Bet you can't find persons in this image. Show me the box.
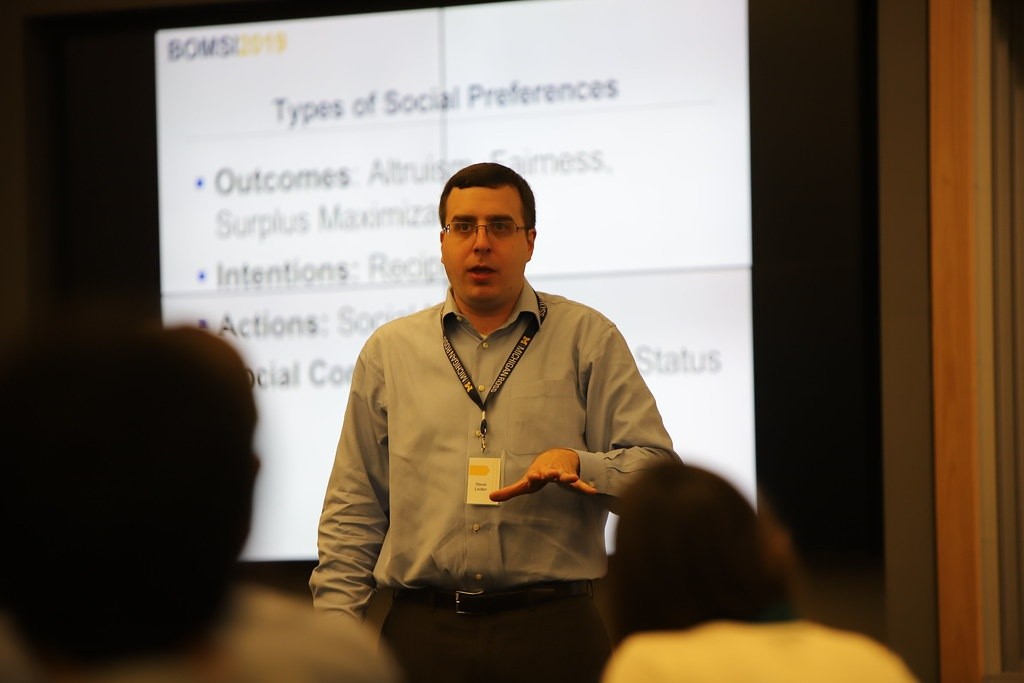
[604,463,915,683]
[309,163,681,683]
[1,299,404,683]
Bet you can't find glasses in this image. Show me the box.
[443,218,533,239]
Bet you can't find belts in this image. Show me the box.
[394,583,588,617]
